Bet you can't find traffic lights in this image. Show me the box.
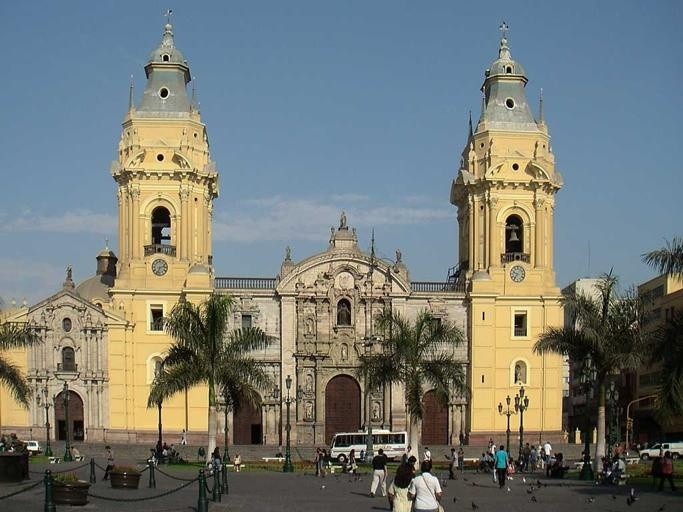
[628,418,633,428]
[654,400,659,409]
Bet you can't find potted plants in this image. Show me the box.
[47,470,92,506]
[105,462,143,490]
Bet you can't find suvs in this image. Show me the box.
[21,440,43,457]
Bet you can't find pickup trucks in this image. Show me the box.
[639,440,682,460]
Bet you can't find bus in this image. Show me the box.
[327,428,411,464]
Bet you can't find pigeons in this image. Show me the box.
[471,502,480,511]
[583,488,642,506]
[507,485,512,493]
[521,475,550,503]
[453,496,458,503]
[473,482,479,487]
[657,504,667,512]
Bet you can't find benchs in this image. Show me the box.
[261,456,285,463]
[225,463,245,472]
[574,459,593,471]
[461,456,480,465]
[330,464,358,475]
[625,457,641,465]
[595,468,629,486]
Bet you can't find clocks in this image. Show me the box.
[509,265,526,283]
[151,259,168,276]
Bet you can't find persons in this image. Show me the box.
[369,449,388,498]
[233,449,243,473]
[71,445,81,455]
[479,437,552,488]
[178,428,187,446]
[315,447,330,474]
[658,451,679,492]
[601,441,647,487]
[210,447,222,473]
[651,450,664,490]
[0,433,29,456]
[342,449,364,474]
[443,447,465,480]
[102,446,115,480]
[148,439,184,467]
[388,445,443,512]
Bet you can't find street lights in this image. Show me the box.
[153,361,167,465]
[605,378,620,459]
[214,382,239,462]
[497,393,517,458]
[34,386,58,457]
[272,372,307,473]
[515,385,531,459]
[60,381,75,461]
